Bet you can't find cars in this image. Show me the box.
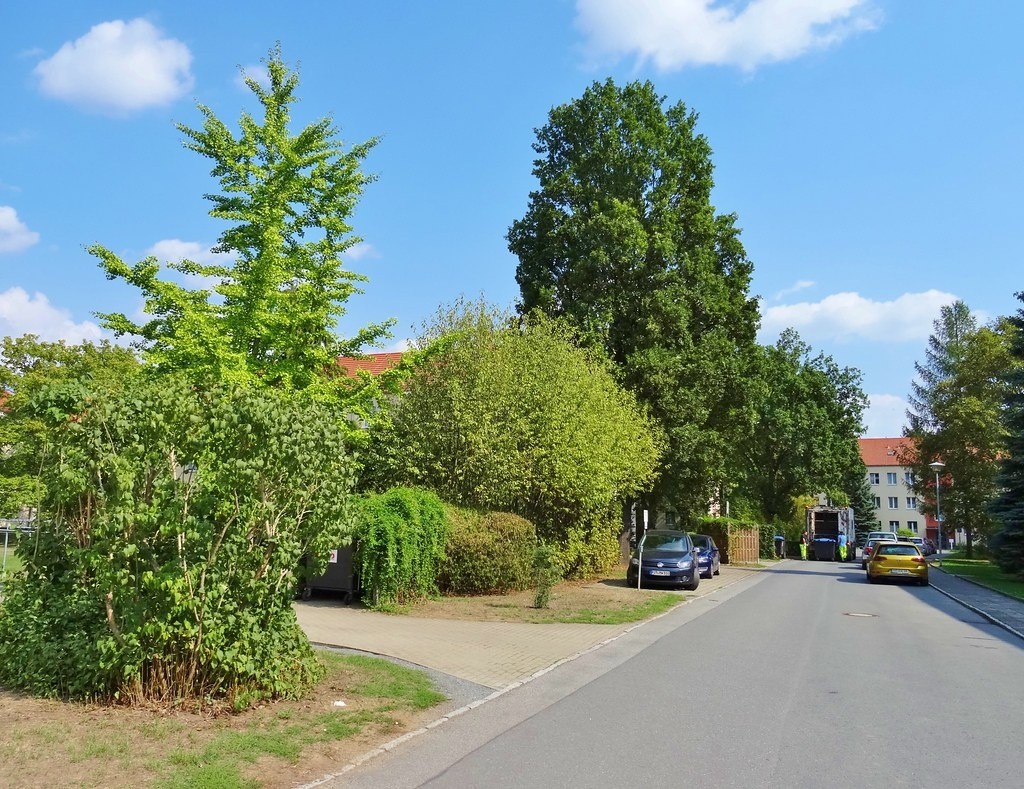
[863,532,898,547]
[674,534,721,579]
[627,530,699,591]
[860,539,897,569]
[896,536,937,556]
[865,541,928,586]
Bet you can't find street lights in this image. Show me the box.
[928,462,946,567]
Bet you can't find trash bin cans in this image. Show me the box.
[774,535,785,557]
[302,538,363,605]
[813,538,836,562]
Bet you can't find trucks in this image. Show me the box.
[805,506,858,561]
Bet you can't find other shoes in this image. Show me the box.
[802,559,808,561]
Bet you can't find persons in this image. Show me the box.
[837,531,847,563]
[799,531,808,561]
